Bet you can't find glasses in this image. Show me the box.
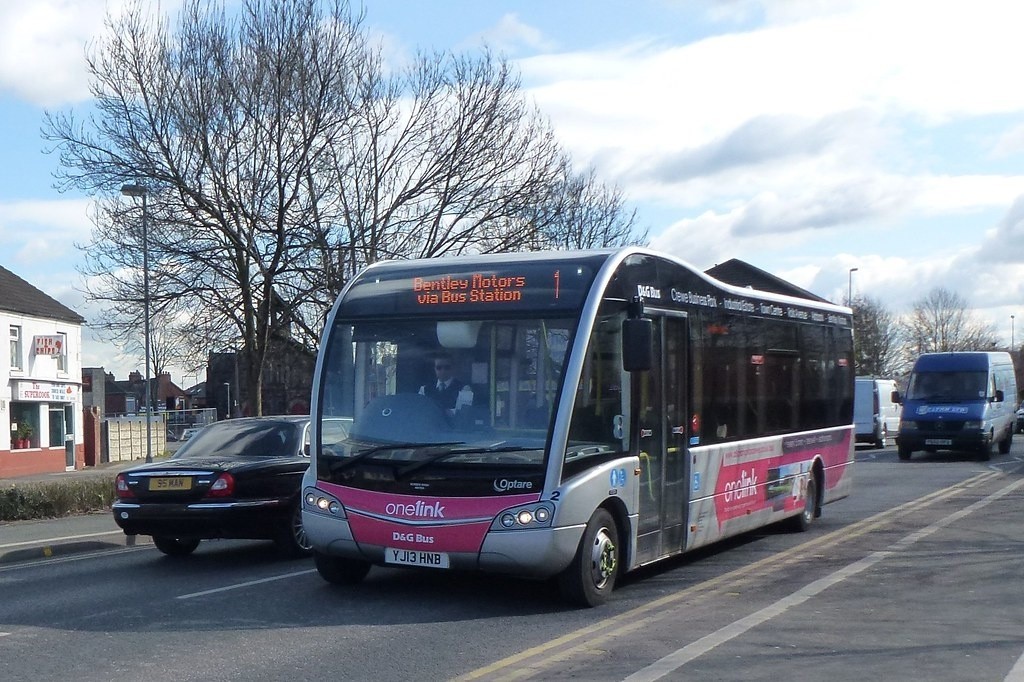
[435,364,451,370]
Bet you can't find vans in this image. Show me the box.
[855,376,903,450]
[890,351,1018,462]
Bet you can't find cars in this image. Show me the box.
[110,416,354,558]
[1017,399,1024,433]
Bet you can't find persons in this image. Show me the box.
[418,354,471,421]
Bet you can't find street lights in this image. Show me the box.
[119,183,155,462]
[223,382,230,419]
[848,266,858,308]
[1010,314,1016,355]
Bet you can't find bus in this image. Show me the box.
[299,244,857,608]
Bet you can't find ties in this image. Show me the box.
[439,382,446,394]
